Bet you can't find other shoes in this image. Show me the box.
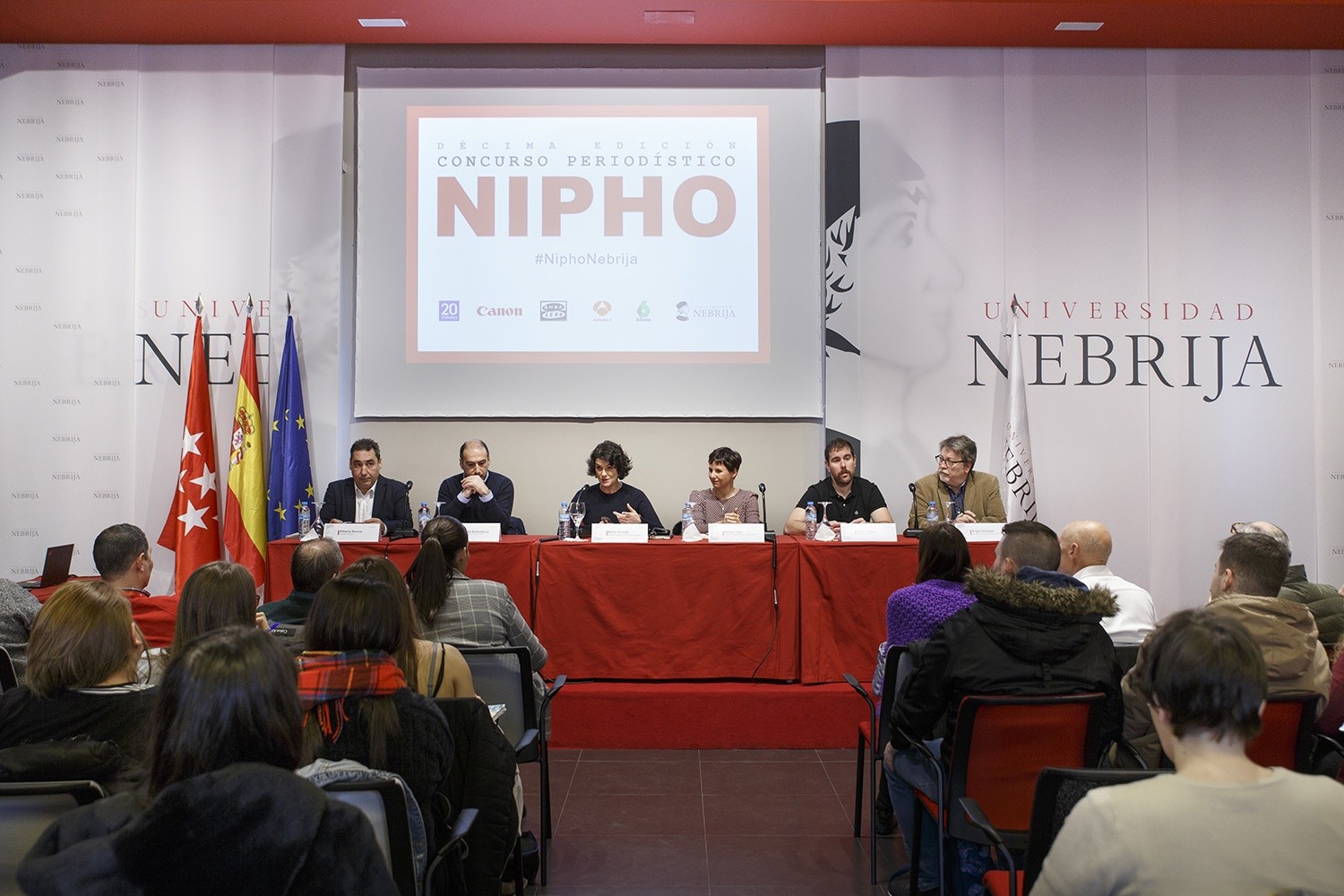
[499,876,528,895]
[876,812,897,835]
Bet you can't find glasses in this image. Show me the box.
[255,594,260,608]
[1231,523,1247,535]
[935,454,969,467]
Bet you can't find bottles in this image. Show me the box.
[682,502,692,539]
[418,502,431,539]
[927,501,939,528]
[298,502,310,539]
[805,501,817,540]
[558,502,570,541]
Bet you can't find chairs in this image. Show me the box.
[0,624,572,896]
[835,642,1344,896]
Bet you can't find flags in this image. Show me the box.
[157,315,220,597]
[224,319,265,588]
[1006,317,1038,524]
[266,315,317,543]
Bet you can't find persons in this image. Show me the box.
[1095,534,1332,786]
[682,447,760,533]
[557,440,666,539]
[0,523,179,764]
[1029,608,1344,896]
[433,439,514,533]
[875,520,1157,896]
[908,434,1007,530]
[256,516,551,740]
[783,437,893,534]
[1314,634,1344,783]
[1230,521,1344,643]
[320,438,414,536]
[17,560,481,896]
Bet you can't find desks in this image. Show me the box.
[259,529,561,675]
[792,525,999,685]
[534,532,802,687]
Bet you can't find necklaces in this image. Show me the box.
[120,587,151,597]
[716,488,735,510]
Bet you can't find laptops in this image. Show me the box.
[17,544,74,588]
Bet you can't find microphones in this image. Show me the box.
[394,481,414,537]
[571,485,589,537]
[904,484,924,536]
[759,483,775,541]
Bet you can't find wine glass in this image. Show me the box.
[310,502,326,538]
[431,502,445,517]
[682,502,702,542]
[817,501,833,530]
[569,502,588,542]
[941,501,956,526]
